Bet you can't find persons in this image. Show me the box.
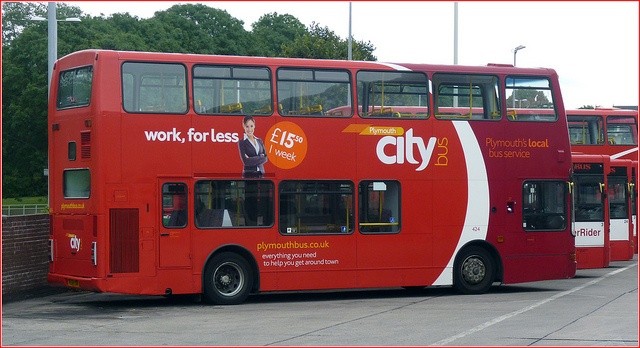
[237,118,268,180]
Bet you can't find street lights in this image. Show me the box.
[512,45,526,108]
[31,2,80,96]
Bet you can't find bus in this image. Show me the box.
[48,49,576,306]
[573,155,610,269]
[610,159,633,262]
[322,105,640,163]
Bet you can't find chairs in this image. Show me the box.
[288,104,324,115]
[490,110,517,118]
[220,102,245,112]
[326,222,350,234]
[253,104,283,113]
[598,137,616,144]
[575,139,584,144]
[192,98,203,114]
[360,108,402,117]
[327,111,343,117]
